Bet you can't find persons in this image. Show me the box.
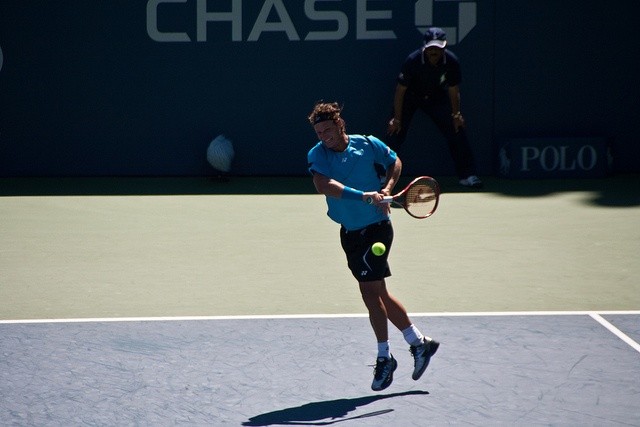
[387,27,483,188]
[305,102,441,391]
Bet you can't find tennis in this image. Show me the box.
[371,241,387,256]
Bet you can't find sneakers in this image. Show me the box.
[409,335,440,380]
[459,175,481,188]
[371,352,398,391]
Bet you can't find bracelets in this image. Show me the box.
[341,184,365,200]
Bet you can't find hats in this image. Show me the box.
[424,27,446,50]
